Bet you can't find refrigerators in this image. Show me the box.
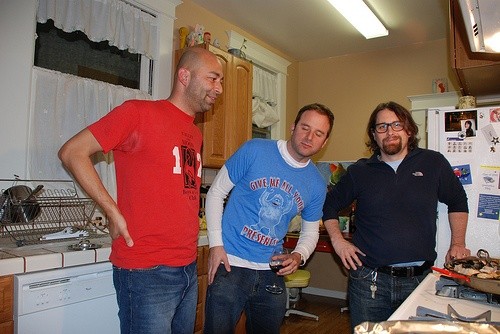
[425,106,499,274]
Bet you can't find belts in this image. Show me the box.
[363,262,434,278]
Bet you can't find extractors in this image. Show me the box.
[459,0,499,53]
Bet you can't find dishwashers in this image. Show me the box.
[13,262,121,334]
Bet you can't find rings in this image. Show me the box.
[463,253,467,256]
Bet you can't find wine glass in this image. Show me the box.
[263,247,288,295]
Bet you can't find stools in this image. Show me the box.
[285,270,319,321]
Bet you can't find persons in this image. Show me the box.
[465,121,473,137]
[58,48,224,334]
[205,104,334,334]
[324,102,468,333]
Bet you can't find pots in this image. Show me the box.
[0,184,44,224]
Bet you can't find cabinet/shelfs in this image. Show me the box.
[176,43,253,169]
[449,0,500,96]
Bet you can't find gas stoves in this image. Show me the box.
[435,266,500,307]
[407,304,500,334]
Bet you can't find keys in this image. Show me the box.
[370,282,377,299]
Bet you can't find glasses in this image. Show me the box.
[375,121,405,133]
